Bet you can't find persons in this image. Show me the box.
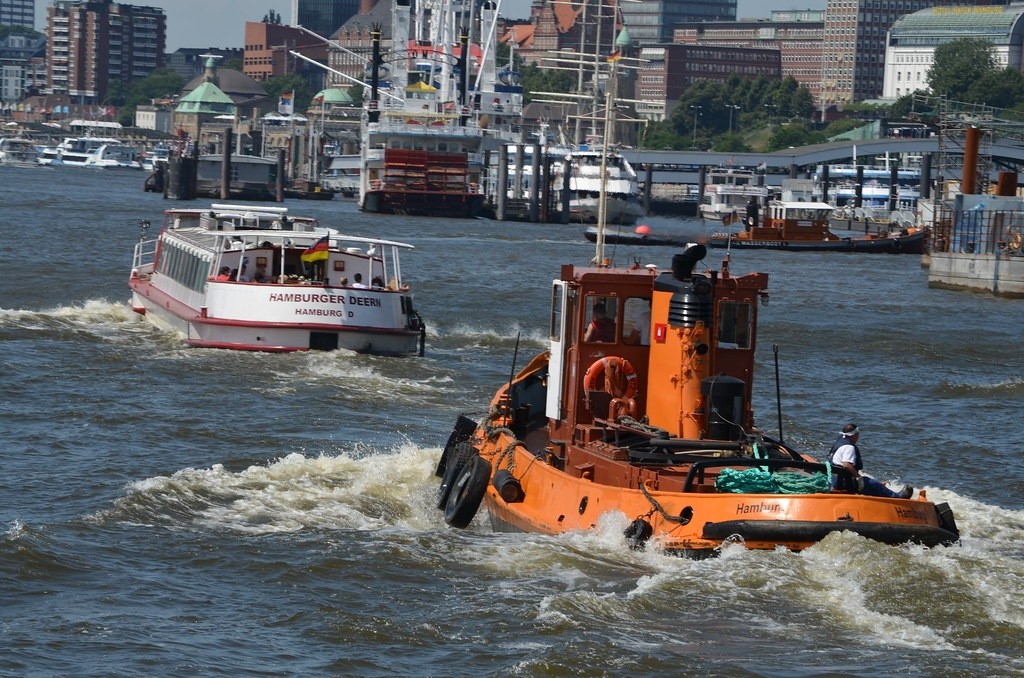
[254,263,271,283]
[216,266,231,281]
[827,424,914,499]
[807,211,815,219]
[239,264,250,282]
[629,301,651,345]
[351,273,368,288]
[228,269,238,281]
[584,304,616,342]
[340,276,348,286]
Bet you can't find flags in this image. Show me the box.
[722,211,739,227]
[607,49,621,63]
[300,236,328,265]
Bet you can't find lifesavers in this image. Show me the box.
[583,356,636,400]
[443,457,493,530]
[437,442,480,511]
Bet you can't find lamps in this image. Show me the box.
[140,219,151,229]
[284,238,295,249]
[367,243,380,258]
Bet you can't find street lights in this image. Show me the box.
[764,104,777,124]
[690,104,702,142]
[724,103,740,134]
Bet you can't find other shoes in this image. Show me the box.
[898,484,913,499]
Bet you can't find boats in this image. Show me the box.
[284,179,333,200]
[435,92,959,565]
[584,200,930,253]
[289,0,647,225]
[699,162,770,222]
[129,203,426,357]
[0,120,277,198]
